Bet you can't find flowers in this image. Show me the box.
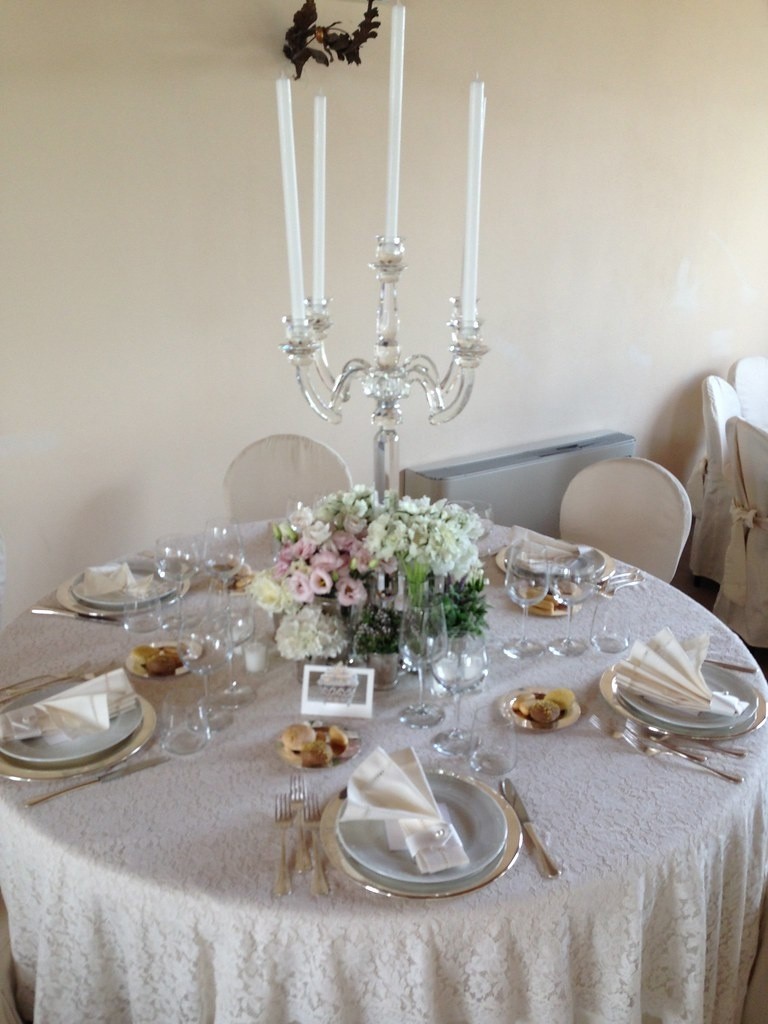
[244,487,491,657]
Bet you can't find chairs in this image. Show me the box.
[558,353,768,649]
[223,433,352,527]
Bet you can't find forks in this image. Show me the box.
[0,658,116,706]
[587,567,645,599]
[589,713,745,783]
[273,774,330,895]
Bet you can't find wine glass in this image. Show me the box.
[427,630,486,756]
[499,541,587,659]
[158,519,256,735]
[395,597,449,730]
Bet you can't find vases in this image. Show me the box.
[297,655,327,685]
[365,652,399,690]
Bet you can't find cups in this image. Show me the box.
[122,582,165,637]
[592,598,632,654]
[470,707,517,776]
[160,686,210,759]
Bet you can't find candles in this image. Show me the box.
[383,0,406,240]
[274,69,305,330]
[312,89,328,312]
[460,71,488,336]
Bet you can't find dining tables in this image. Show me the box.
[0,517,767,1024]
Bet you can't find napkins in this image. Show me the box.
[0,668,138,745]
[610,626,750,718]
[510,525,592,574]
[339,746,470,874]
[81,562,157,600]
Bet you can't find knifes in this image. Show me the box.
[32,606,122,626]
[23,756,170,805]
[502,778,560,879]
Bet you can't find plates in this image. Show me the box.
[502,689,580,728]
[320,771,522,899]
[55,558,191,617]
[599,659,766,739]
[126,638,202,676]
[0,681,157,785]
[277,720,362,770]
[495,540,616,583]
